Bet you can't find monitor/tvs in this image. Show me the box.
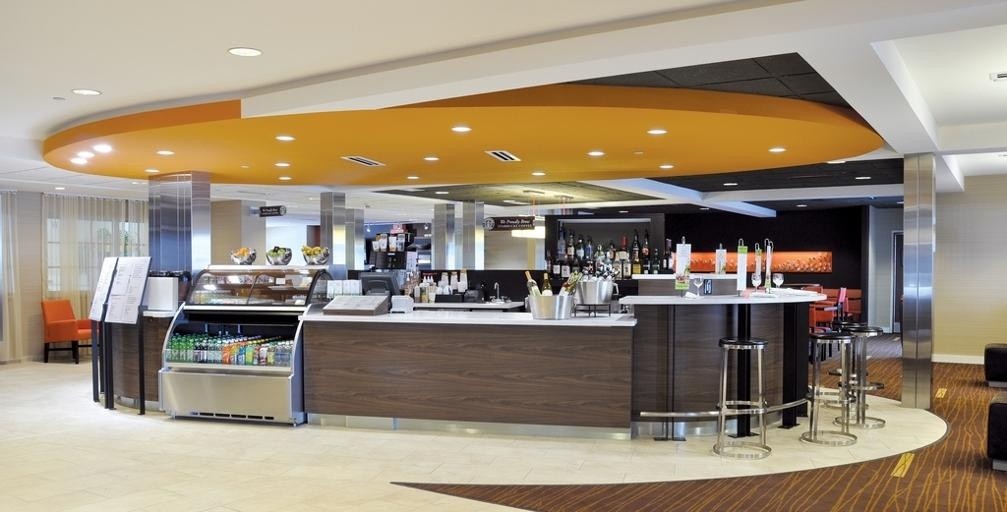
[360,272,401,295]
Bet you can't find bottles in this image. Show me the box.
[524,270,540,296]
[194,289,231,304]
[543,229,672,280]
[559,271,582,296]
[413,268,467,304]
[541,273,552,296]
[166,330,292,368]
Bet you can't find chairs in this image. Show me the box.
[802,285,862,361]
[39,298,100,364]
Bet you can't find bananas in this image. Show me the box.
[302,245,328,255]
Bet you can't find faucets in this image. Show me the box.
[494,281,501,298]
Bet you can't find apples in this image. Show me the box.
[270,249,285,256]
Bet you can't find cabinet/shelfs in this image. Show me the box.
[157,264,332,425]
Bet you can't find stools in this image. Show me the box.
[712,320,885,460]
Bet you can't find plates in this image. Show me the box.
[268,285,309,291]
[159,270,184,277]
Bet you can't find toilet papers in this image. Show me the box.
[755,250,762,277]
[736,245,748,290]
[675,242,691,291]
[715,249,727,275]
[765,245,772,288]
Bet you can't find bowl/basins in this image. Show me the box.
[527,294,574,320]
[266,248,292,265]
[231,248,256,265]
[302,254,330,265]
[575,282,612,304]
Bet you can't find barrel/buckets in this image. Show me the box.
[530,293,575,318]
[575,279,619,305]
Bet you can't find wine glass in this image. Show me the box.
[771,273,783,290]
[751,272,762,292]
[693,273,703,298]
